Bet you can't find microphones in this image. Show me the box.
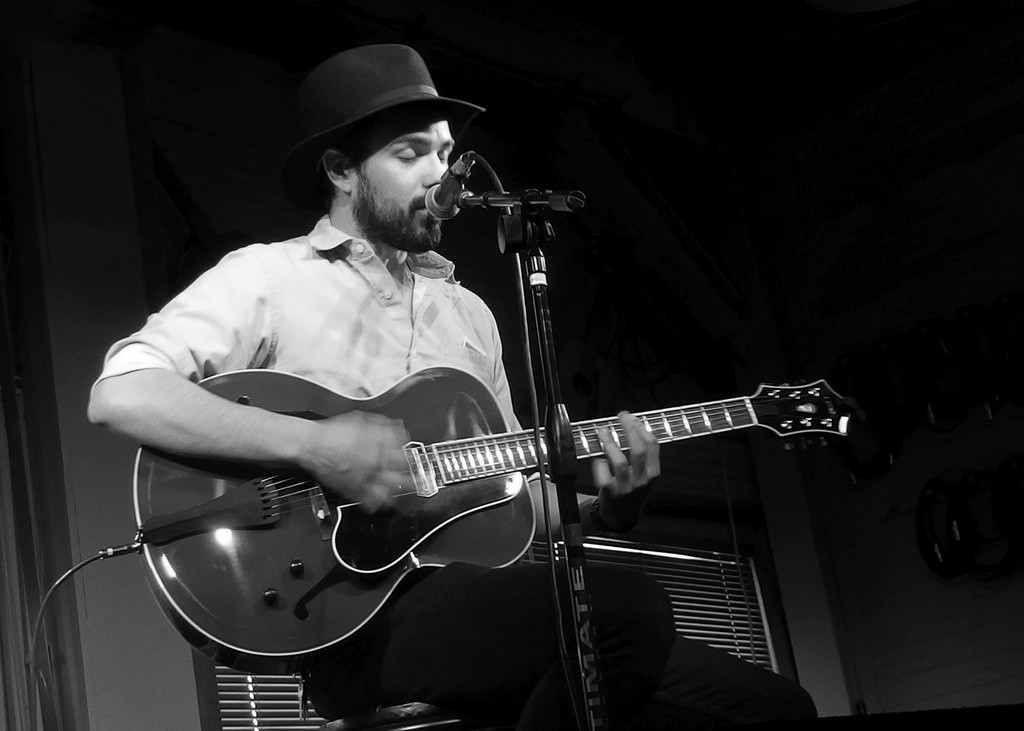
[425,151,477,219]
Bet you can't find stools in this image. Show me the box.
[325,702,460,731]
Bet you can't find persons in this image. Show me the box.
[87,44,660,731]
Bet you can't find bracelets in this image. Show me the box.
[590,498,640,536]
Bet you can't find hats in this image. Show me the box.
[285,44,486,167]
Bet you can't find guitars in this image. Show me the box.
[133,372,853,669]
